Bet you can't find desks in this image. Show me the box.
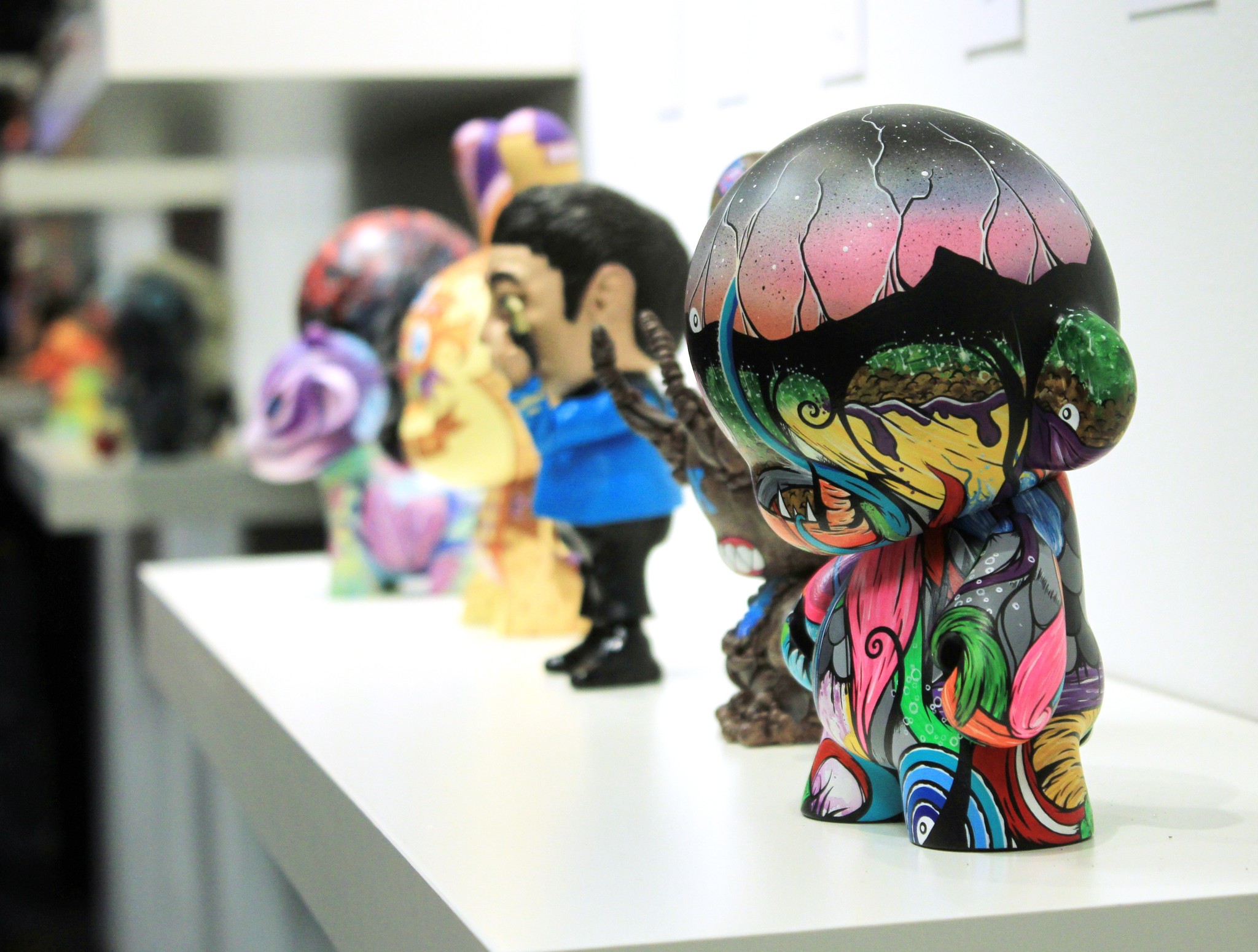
[133,553,1258,952]
[7,424,332,952]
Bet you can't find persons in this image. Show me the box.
[482,181,690,688]
